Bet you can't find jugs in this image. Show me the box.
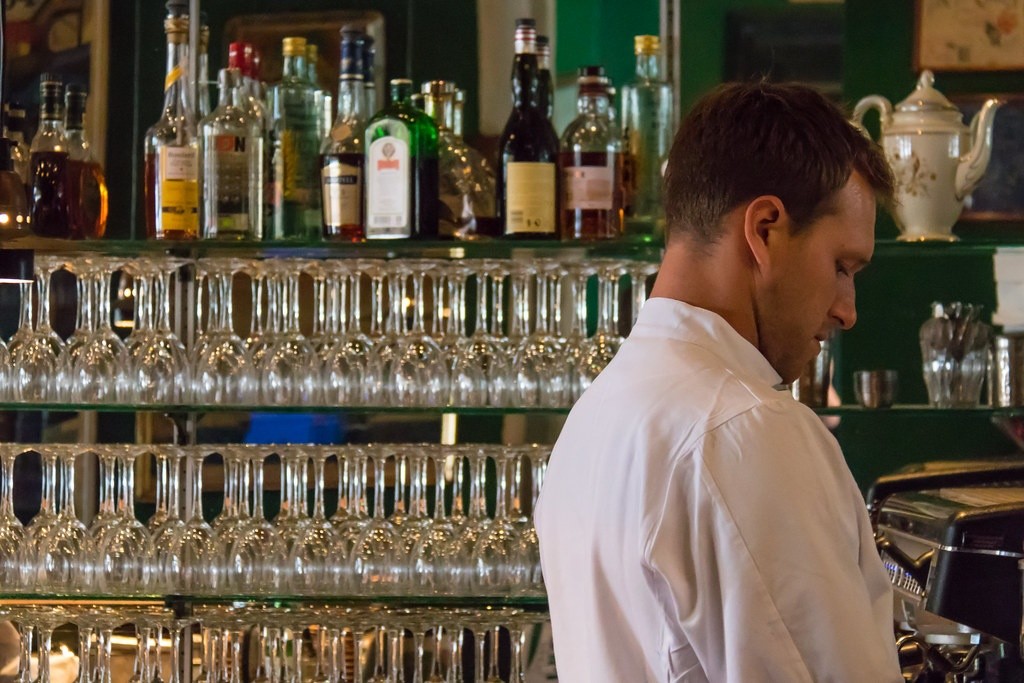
[851,68,1006,244]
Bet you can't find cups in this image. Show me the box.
[853,370,901,408]
[790,336,830,411]
[993,334,1023,408]
[917,299,991,411]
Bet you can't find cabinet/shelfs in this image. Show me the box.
[1,236,671,615]
[806,235,1024,418]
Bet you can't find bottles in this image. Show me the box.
[0,15,676,248]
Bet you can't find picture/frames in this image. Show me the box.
[719,7,843,102]
[910,0,1024,80]
[0,0,108,164]
[950,92,1024,224]
[223,12,385,112]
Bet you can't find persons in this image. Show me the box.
[533,82,903,683]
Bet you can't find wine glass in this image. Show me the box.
[0,252,659,683]
